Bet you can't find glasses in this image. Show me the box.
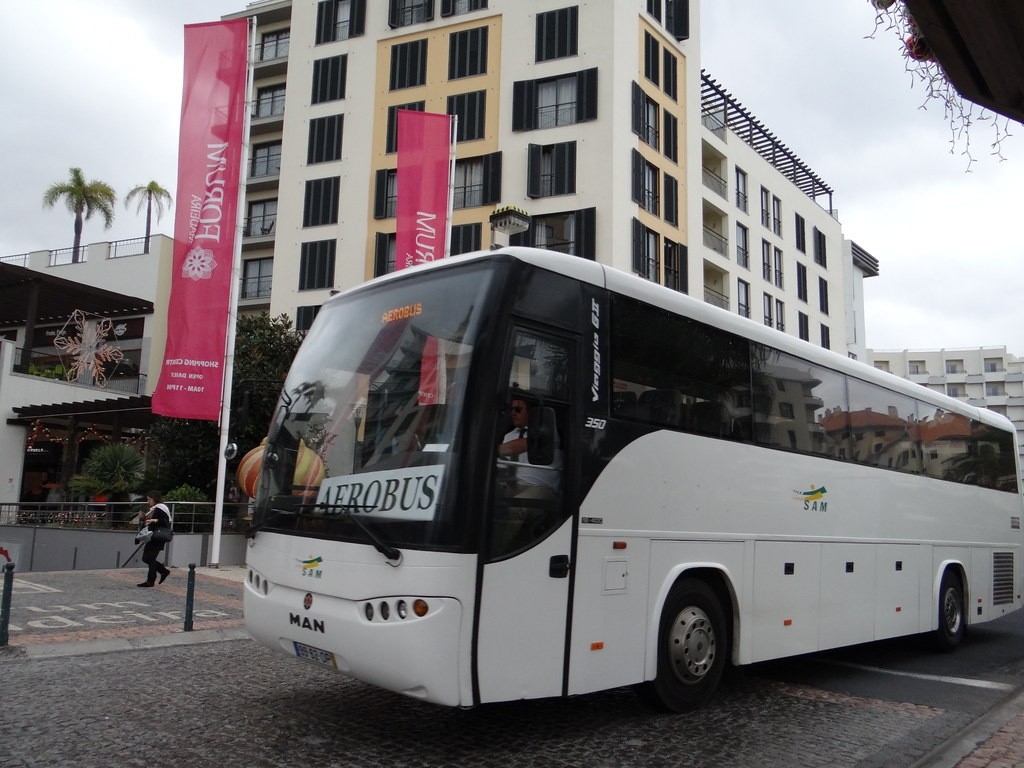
[509,404,526,414]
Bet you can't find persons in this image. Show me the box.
[493,397,565,556]
[136,490,174,588]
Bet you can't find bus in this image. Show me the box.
[242,243,1022,714]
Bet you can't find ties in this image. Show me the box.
[507,428,527,490]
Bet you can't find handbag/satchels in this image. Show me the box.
[154,528,174,541]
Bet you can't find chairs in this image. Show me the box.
[607,384,771,442]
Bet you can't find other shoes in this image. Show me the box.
[158,570,170,584]
[137,582,153,587]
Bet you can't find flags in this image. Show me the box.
[396,108,453,409]
[149,18,249,429]
